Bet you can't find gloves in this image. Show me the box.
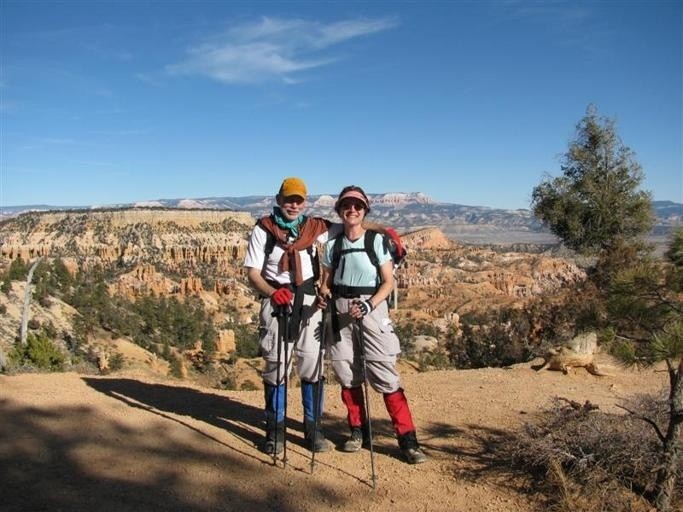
[385,228,403,257]
[272,288,294,305]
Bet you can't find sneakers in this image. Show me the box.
[264,427,285,454]
[344,427,371,452]
[305,430,328,451]
[399,444,426,463]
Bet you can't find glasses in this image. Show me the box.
[341,201,363,210]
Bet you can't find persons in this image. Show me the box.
[316,185,427,465]
[242,177,405,455]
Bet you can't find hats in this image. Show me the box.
[280,178,307,199]
[336,191,368,209]
